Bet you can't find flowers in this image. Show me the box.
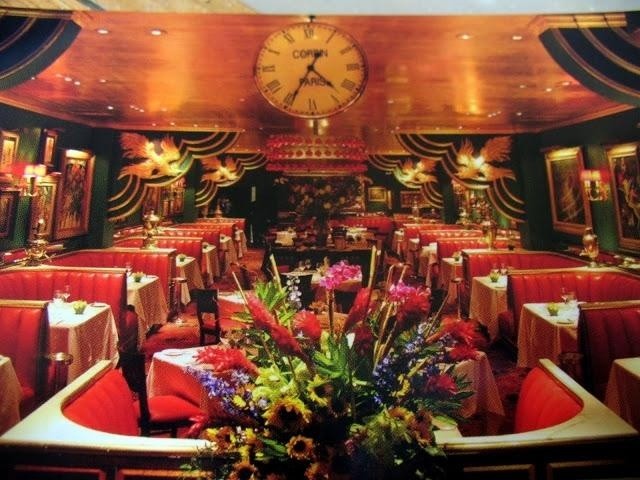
[173,261,491,479]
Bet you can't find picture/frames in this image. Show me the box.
[545,147,593,236]
[606,142,640,251]
[0,130,96,242]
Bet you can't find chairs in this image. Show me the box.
[196,289,249,347]
[116,304,205,438]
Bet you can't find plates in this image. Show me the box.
[162,348,183,356]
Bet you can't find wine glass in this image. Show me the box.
[63,284,72,300]
[53,290,64,305]
[125,262,132,277]
[561,287,575,304]
[305,259,312,271]
[298,260,305,273]
[316,262,323,271]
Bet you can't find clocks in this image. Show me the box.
[253,15,368,120]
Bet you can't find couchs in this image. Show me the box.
[0,357,639,480]
